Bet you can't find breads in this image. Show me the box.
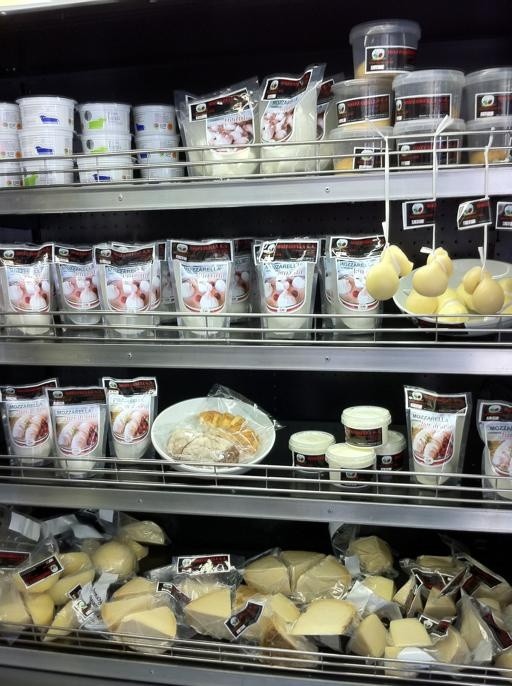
[171,407,261,466]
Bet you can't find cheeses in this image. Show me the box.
[0,521,512,683]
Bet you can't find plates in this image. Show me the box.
[11,278,380,320]
[207,109,328,152]
[408,427,512,479]
[11,410,151,459]
[392,257,512,333]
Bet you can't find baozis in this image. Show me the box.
[7,267,374,310]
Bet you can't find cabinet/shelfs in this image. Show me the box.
[1,129,512,686]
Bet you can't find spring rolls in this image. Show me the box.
[12,406,147,452]
[413,426,512,474]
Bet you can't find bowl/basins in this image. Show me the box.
[150,398,279,476]
